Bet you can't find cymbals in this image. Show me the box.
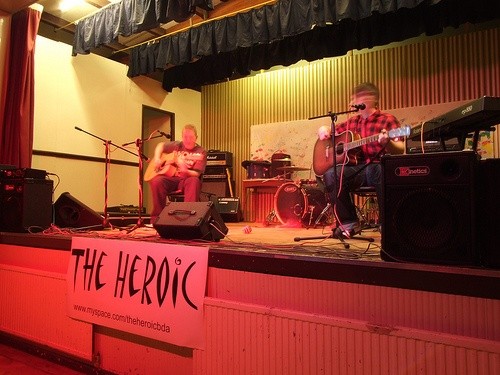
[274,167,311,171]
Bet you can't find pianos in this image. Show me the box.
[408,96,500,151]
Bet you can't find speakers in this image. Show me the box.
[52,192,104,231]
[0,178,53,232]
[380,149,479,267]
[153,201,230,242]
[209,194,242,222]
[198,177,229,202]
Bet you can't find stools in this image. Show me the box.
[352,185,380,234]
[170,191,185,201]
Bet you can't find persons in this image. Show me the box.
[149,124,207,224]
[318,82,404,236]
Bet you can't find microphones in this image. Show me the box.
[157,130,171,139]
[350,103,365,110]
[142,153,150,163]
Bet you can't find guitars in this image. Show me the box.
[144,153,204,182]
[313,124,409,178]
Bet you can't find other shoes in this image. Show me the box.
[341,223,362,237]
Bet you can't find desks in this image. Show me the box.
[242,178,294,222]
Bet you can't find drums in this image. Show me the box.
[273,182,324,226]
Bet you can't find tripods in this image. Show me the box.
[74,127,162,234]
[293,108,375,249]
